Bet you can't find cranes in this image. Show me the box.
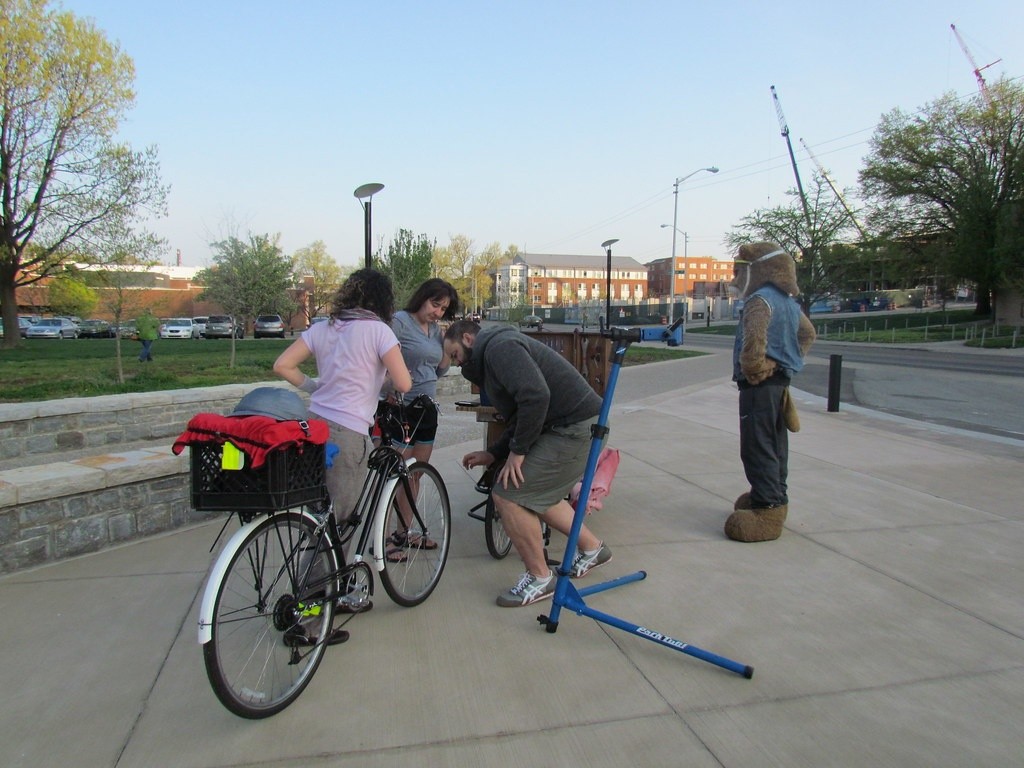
[951,23,1003,117]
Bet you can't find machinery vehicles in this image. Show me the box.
[768,84,874,239]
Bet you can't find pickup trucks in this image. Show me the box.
[849,291,894,310]
[953,288,969,297]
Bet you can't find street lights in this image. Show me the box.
[669,167,719,324]
[660,223,688,333]
[532,272,538,316]
[472,251,494,313]
[464,276,478,313]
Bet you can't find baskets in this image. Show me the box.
[189,442,325,513]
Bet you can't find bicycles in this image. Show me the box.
[201,387,455,718]
[468,425,552,560]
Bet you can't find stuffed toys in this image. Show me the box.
[724,242,817,542]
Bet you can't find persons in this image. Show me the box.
[368,279,460,562]
[236,324,244,339]
[193,324,200,339]
[273,270,412,647]
[582,312,589,328]
[442,320,613,607]
[135,308,161,364]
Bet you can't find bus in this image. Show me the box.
[20,316,42,325]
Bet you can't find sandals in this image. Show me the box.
[391,530,437,550]
[369,539,407,562]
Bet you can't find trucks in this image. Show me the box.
[915,285,938,293]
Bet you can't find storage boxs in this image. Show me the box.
[188,437,327,514]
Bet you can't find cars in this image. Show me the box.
[159,318,201,339]
[78,318,159,340]
[0,317,32,339]
[26,316,83,339]
[518,316,544,328]
[192,316,209,337]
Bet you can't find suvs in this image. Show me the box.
[253,314,285,339]
[467,312,483,323]
[205,314,245,339]
[311,316,329,327]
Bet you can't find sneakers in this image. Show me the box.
[497,570,557,607]
[572,541,612,577]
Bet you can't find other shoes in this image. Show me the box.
[333,597,373,613]
[284,629,350,646]
